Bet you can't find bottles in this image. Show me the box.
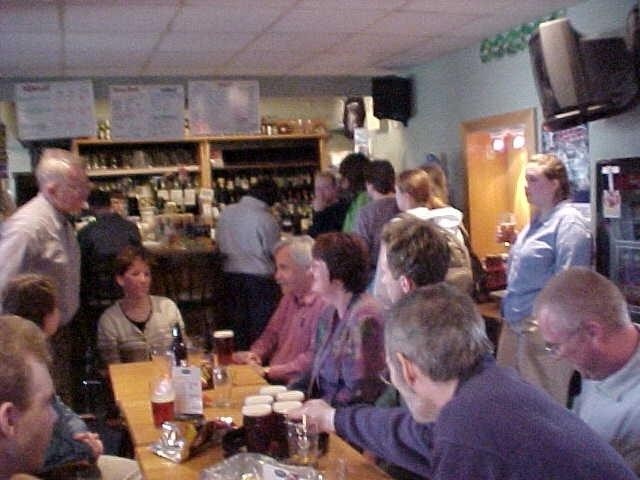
[297,118,313,134]
[241,384,330,461]
[97,119,111,140]
[98,177,199,217]
[169,323,187,367]
[298,211,311,233]
[267,124,273,136]
[165,218,178,249]
[281,210,293,234]
[259,117,266,135]
[84,151,117,170]
[215,171,313,208]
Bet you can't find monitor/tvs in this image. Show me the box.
[527,17,639,131]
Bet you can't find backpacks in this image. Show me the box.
[398,209,473,294]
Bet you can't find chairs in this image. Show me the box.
[162,252,226,314]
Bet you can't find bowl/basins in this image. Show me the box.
[489,290,508,307]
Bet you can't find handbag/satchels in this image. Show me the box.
[473,256,490,303]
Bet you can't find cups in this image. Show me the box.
[212,329,236,367]
[150,391,176,430]
[212,366,234,408]
[151,342,171,375]
[287,421,320,468]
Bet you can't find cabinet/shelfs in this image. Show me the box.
[71,134,329,220]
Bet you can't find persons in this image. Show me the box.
[95,246,191,365]
[1,274,141,478]
[77,188,143,352]
[240,151,474,479]
[216,177,281,352]
[496,153,595,408]
[1,147,97,408]
[286,282,636,480]
[532,265,639,471]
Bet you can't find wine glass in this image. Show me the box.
[500,212,516,250]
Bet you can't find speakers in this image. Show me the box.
[372,74,412,127]
